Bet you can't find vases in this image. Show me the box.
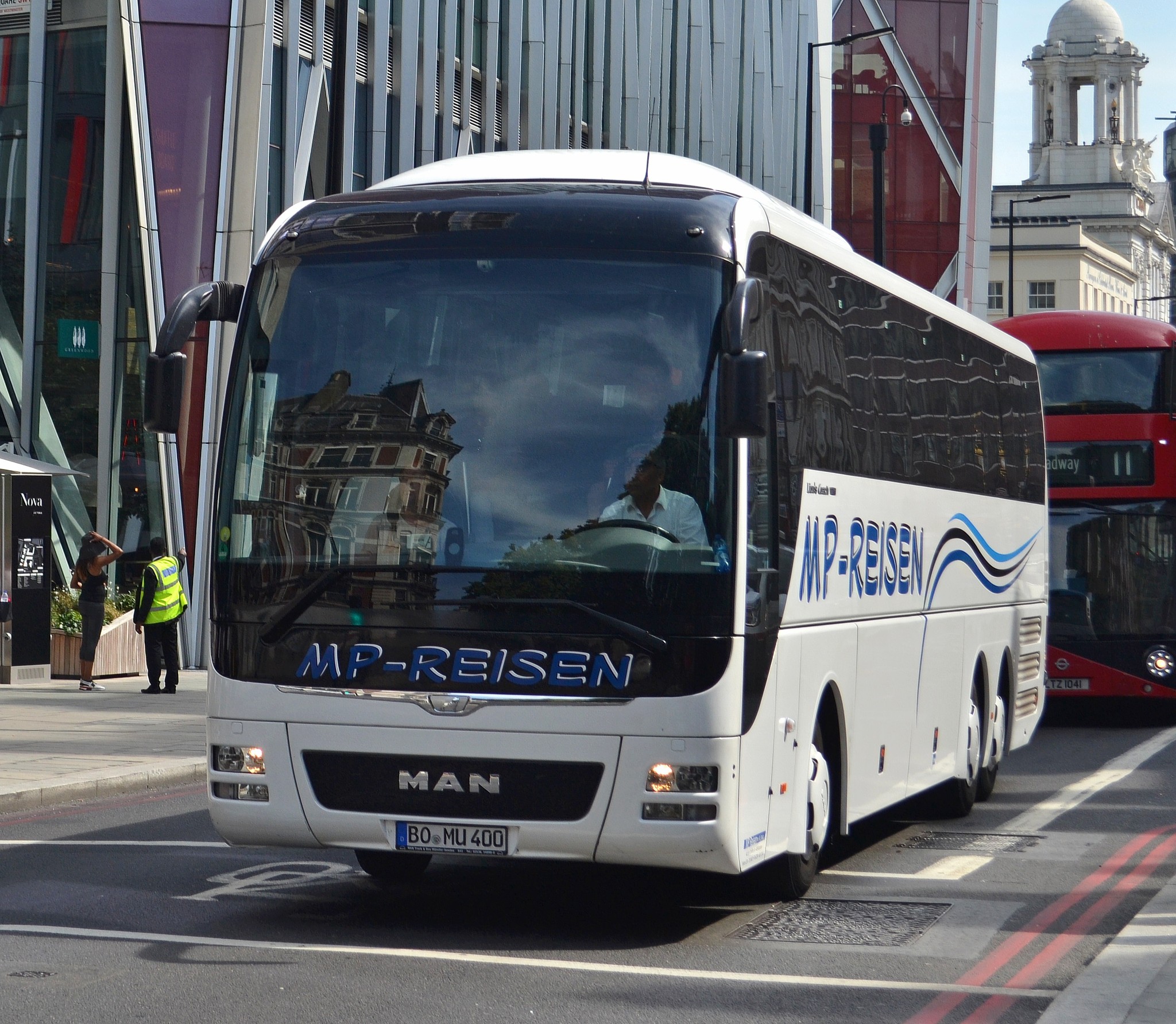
[49,609,150,677]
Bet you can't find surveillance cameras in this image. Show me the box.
[901,111,912,126]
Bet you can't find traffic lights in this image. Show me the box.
[349,594,367,628]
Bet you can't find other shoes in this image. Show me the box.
[141,685,162,694]
[161,685,176,693]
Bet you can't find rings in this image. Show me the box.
[137,630,139,631]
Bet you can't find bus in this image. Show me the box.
[987,311,1176,700]
[143,149,1049,898]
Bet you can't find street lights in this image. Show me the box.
[803,27,895,217]
[1134,295,1176,317]
[1008,195,1070,317]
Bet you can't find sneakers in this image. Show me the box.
[79,679,105,691]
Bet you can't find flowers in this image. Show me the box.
[52,579,138,634]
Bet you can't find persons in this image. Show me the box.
[70,531,123,690]
[133,537,189,694]
[598,444,709,548]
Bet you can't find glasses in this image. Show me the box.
[626,457,659,471]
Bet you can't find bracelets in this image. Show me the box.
[101,537,104,541]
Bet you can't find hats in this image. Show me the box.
[79,533,107,560]
